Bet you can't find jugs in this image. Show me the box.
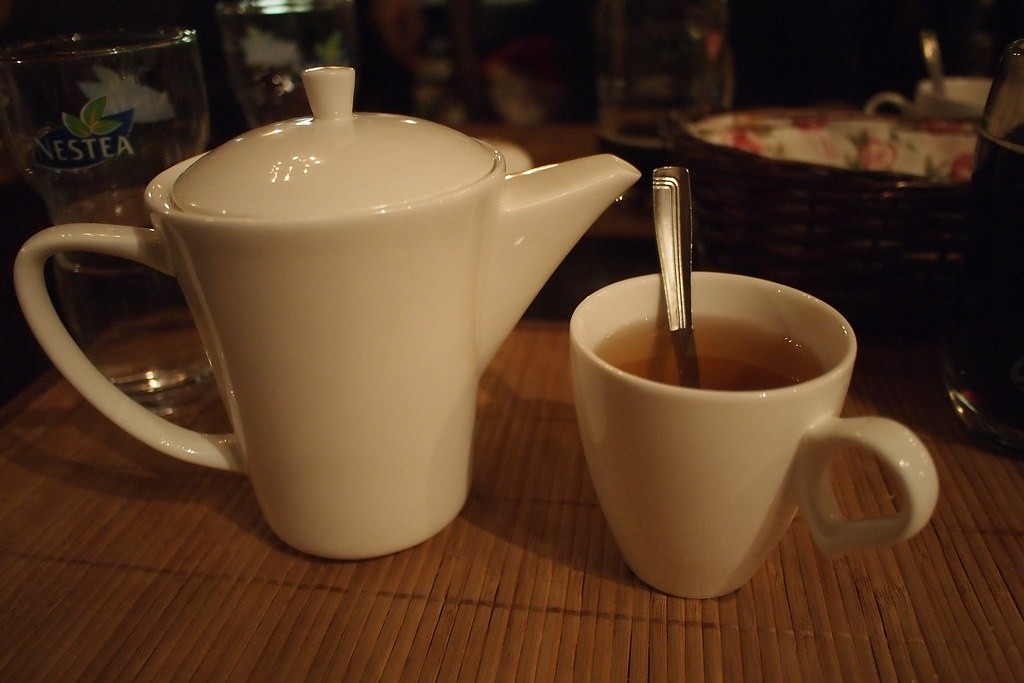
[13,64,642,561]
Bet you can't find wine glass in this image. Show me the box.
[949,41,1024,452]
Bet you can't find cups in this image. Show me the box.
[568,272,938,599]
[860,71,994,123]
[596,0,734,183]
[0,30,212,396]
[213,1,358,128]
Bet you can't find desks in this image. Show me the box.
[0,117,1024,683]
